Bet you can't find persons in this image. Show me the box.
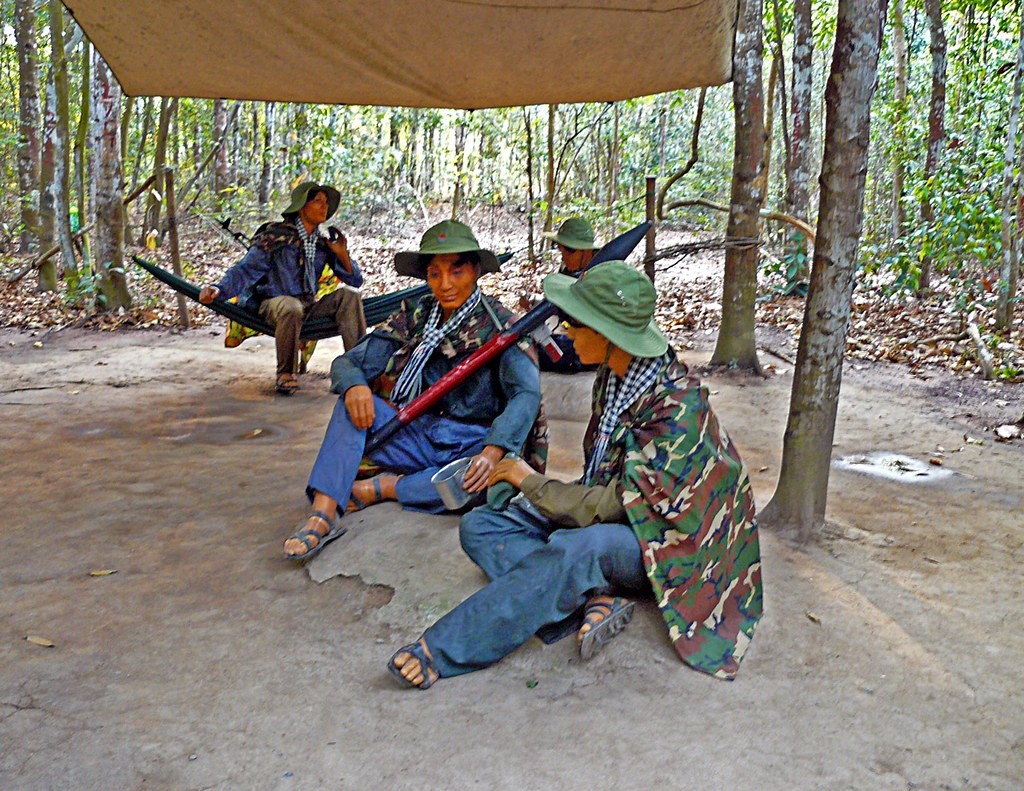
[521,219,600,374]
[198,182,367,390]
[388,260,762,688]
[282,220,548,561]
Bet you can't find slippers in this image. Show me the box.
[388,634,439,690]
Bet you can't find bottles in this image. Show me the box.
[533,324,563,363]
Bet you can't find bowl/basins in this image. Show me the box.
[431,458,480,509]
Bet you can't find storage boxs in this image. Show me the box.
[541,333,599,374]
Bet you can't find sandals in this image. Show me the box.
[283,510,347,559]
[578,597,635,662]
[347,473,398,515]
[276,371,300,390]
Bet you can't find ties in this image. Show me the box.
[580,357,665,485]
[389,286,482,411]
[296,217,319,295]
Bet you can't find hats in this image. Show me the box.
[541,218,603,250]
[544,261,669,358]
[281,181,340,220]
[394,220,503,278]
